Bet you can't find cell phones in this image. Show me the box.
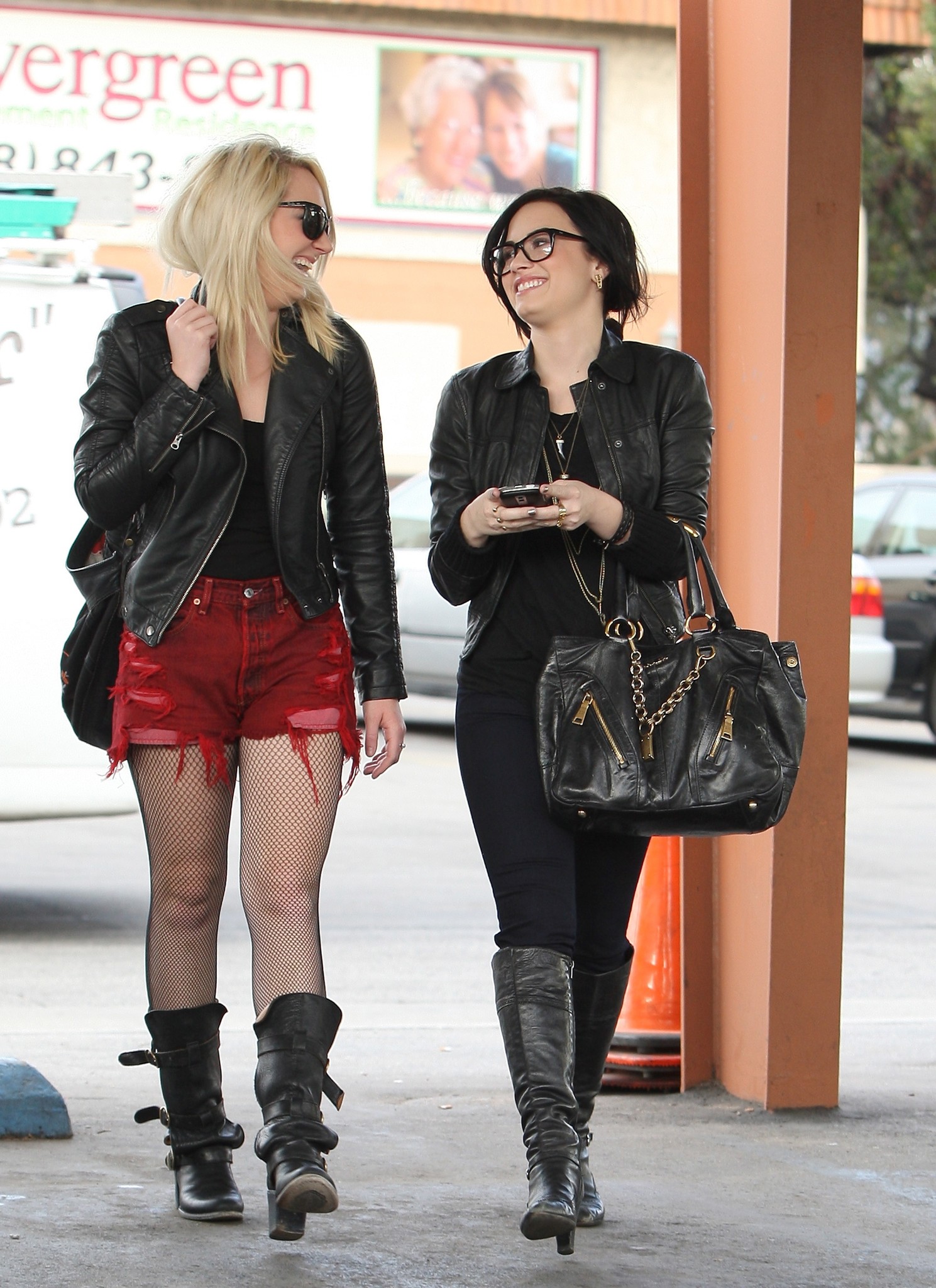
[498,483,553,507]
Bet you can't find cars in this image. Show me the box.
[389,463,898,705]
[847,480,936,739]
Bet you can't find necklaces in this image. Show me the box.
[549,379,588,440]
[547,378,588,480]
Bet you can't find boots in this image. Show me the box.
[118,998,245,1220]
[252,993,345,1241]
[492,945,584,1255]
[574,938,634,1226]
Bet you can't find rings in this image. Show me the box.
[399,743,406,749]
[493,505,507,531]
[556,505,566,527]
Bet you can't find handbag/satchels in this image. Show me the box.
[60,588,124,752]
[537,515,805,836]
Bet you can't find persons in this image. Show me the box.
[427,187,809,1255]
[383,56,578,212]
[59,132,406,1242]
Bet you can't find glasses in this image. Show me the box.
[488,228,590,277]
[277,201,332,240]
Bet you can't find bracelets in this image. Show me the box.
[592,500,633,548]
